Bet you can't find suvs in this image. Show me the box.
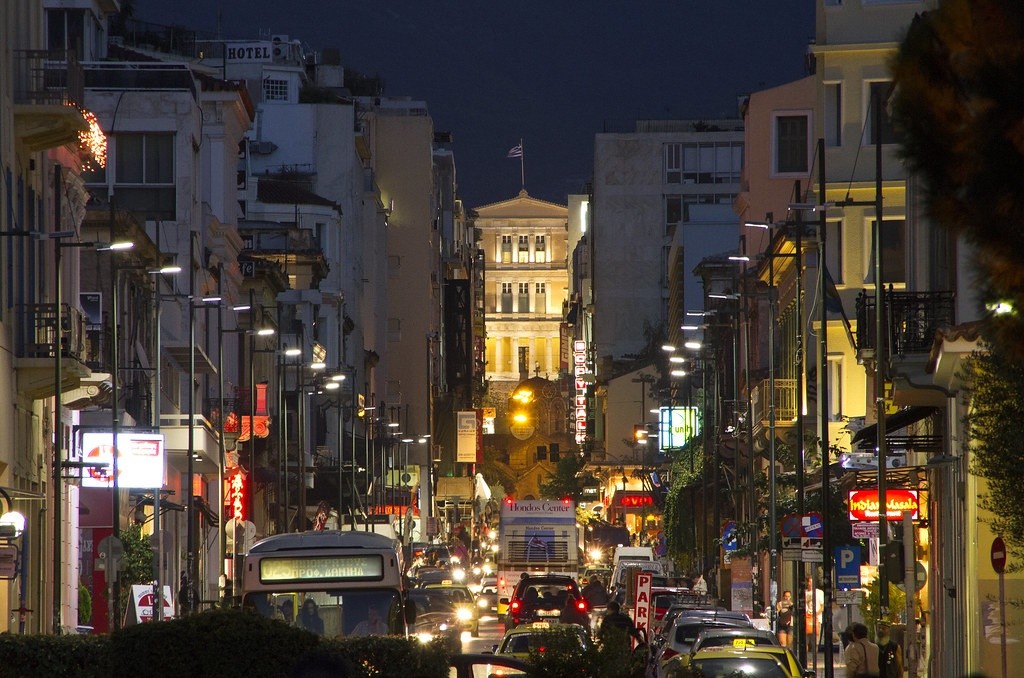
[500,575,590,635]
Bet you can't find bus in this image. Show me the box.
[493,500,584,622]
[241,529,416,640]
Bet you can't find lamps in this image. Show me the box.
[193,452,202,462]
[0,487,25,539]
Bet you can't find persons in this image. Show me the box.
[178,569,388,637]
[754,575,906,678]
[559,575,647,652]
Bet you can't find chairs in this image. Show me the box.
[453,591,464,601]
[557,590,567,597]
[318,606,342,638]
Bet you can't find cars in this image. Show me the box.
[481,622,598,678]
[445,654,533,678]
[581,569,613,591]
[608,547,817,678]
[403,542,499,653]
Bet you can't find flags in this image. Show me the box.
[508,144,522,158]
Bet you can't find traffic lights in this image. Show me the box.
[879,540,905,582]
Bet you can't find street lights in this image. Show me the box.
[53,240,134,634]
[188,304,345,619]
[662,293,778,634]
[745,220,835,678]
[728,253,808,668]
[337,406,431,532]
[154,294,222,620]
[788,199,893,622]
[110,264,181,631]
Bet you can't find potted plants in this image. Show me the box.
[78,585,94,635]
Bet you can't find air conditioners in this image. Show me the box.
[400,472,418,486]
[271,35,288,59]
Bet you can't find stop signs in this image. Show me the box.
[991,538,1006,573]
[139,594,173,624]
